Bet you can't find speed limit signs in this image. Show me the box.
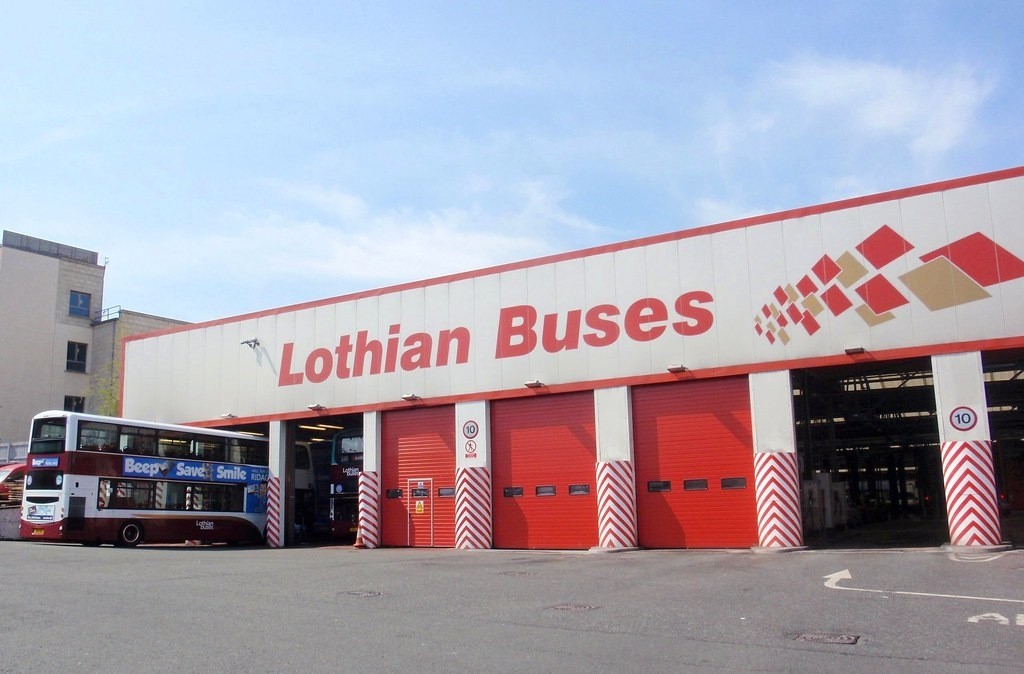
[951,406,977,430]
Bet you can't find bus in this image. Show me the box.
[17,408,319,548]
[326,424,364,534]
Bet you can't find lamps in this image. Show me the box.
[667,364,687,372]
[525,379,543,387]
[402,394,420,400]
[220,413,236,418]
[845,347,863,354]
[308,404,325,410]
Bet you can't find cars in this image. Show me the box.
[0,463,26,502]
